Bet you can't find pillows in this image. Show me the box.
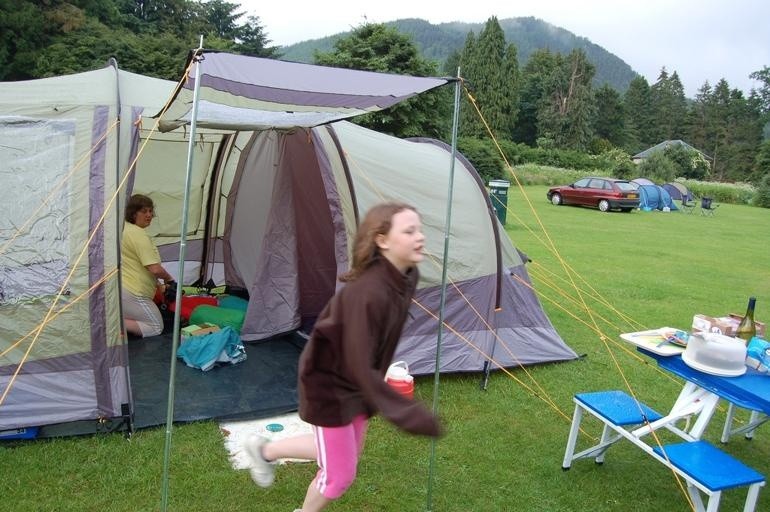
[163,292,248,336]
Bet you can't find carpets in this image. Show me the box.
[217,408,322,469]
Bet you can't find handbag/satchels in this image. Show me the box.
[176,325,248,371]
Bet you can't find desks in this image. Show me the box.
[636,325,770,449]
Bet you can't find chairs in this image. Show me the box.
[679,196,698,216]
[701,196,720,216]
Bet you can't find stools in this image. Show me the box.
[719,401,770,445]
[560,388,657,489]
[645,435,768,512]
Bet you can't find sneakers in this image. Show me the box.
[243,434,274,487]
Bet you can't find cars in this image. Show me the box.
[546,177,641,212]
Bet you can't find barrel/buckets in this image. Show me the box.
[383,360,415,402]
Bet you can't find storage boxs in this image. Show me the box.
[690,311,764,340]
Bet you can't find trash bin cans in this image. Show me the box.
[489,180,509,225]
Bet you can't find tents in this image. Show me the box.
[0,44,586,442]
[628,176,701,212]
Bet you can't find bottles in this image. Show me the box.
[734,296,758,343]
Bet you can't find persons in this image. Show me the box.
[247,200,444,511]
[121,192,173,337]
